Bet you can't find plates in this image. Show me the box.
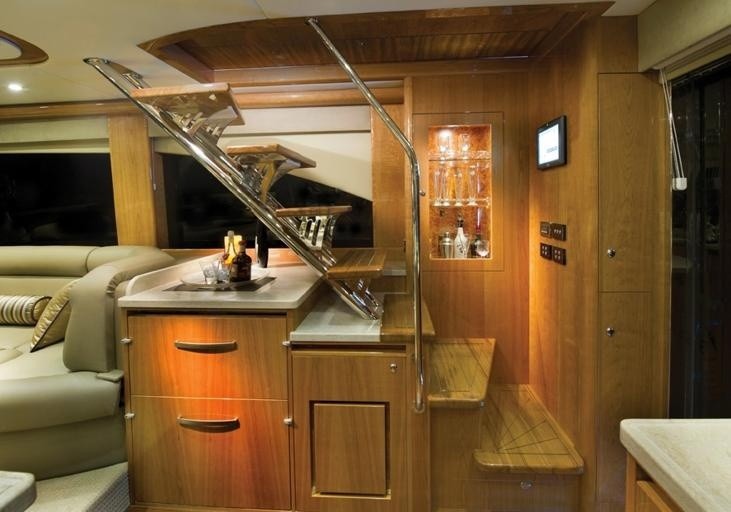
[177,265,268,288]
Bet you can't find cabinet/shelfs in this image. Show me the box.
[120,308,287,512]
[292,351,407,512]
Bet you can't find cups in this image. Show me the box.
[198,258,231,284]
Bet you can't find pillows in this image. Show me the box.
[30,278,80,353]
[1,294,52,326]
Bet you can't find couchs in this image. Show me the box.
[1,245,175,480]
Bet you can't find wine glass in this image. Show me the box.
[474,238,490,258]
[429,130,473,160]
[430,163,487,204]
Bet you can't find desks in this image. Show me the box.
[0,471,36,512]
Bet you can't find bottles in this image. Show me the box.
[437,209,471,259]
[229,240,230,241]
[230,240,254,282]
[225,231,238,265]
